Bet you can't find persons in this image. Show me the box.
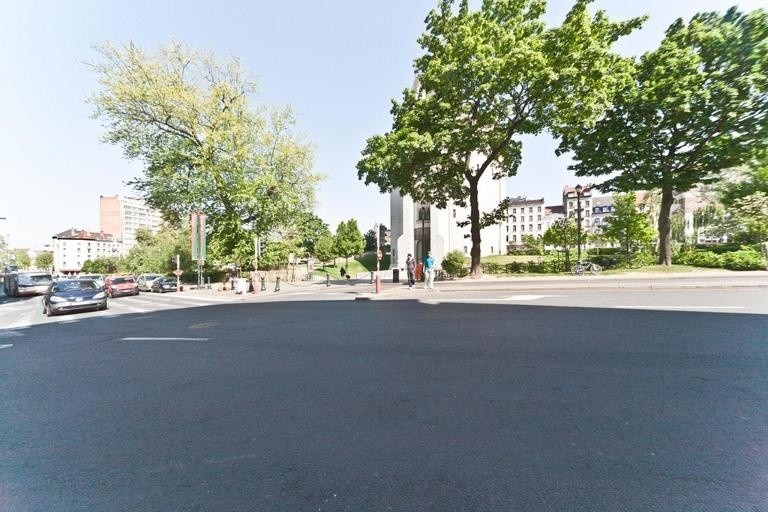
[405,254,416,289]
[423,251,435,289]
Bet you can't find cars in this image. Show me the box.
[42,271,184,317]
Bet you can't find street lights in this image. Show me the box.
[575,184,583,266]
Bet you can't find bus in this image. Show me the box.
[4,270,53,297]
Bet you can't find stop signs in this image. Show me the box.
[376,250,383,262]
